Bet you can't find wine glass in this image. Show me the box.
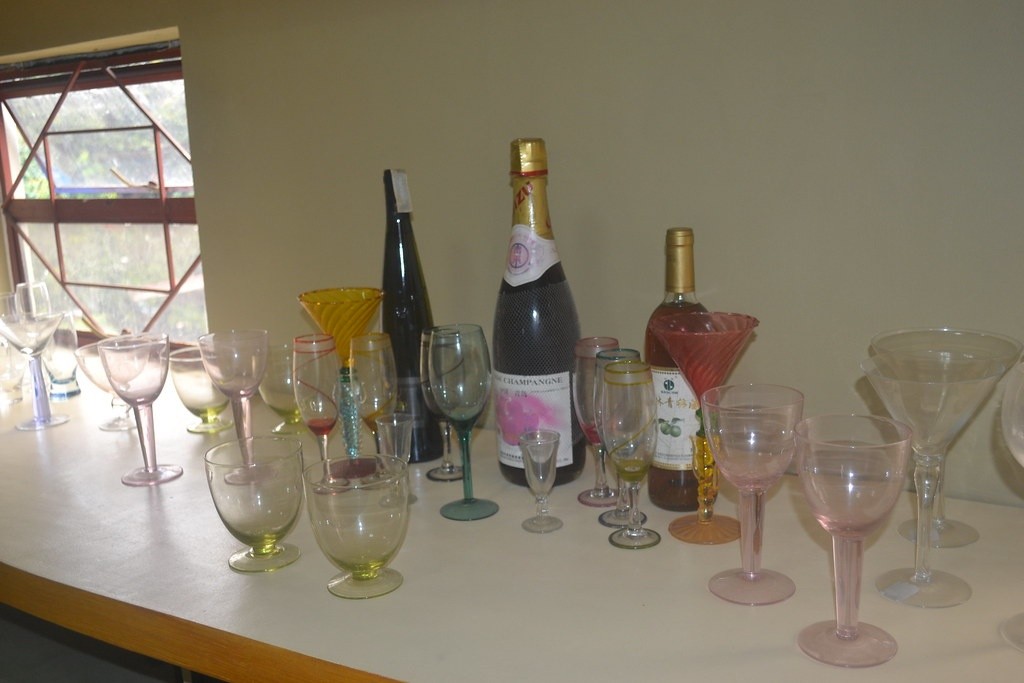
[859,348,1005,609]
[699,383,805,608]
[792,414,912,671]
[0,281,416,600]
[571,336,625,506]
[647,310,761,548]
[594,348,643,529]
[604,361,662,549]
[1001,358,1023,651]
[871,329,1024,549]
[419,322,500,521]
[514,428,564,534]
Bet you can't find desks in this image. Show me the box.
[0,347,1024,683]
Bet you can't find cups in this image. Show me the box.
[39,311,81,397]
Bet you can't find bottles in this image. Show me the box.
[644,226,719,512]
[490,136,587,486]
[381,168,445,465]
